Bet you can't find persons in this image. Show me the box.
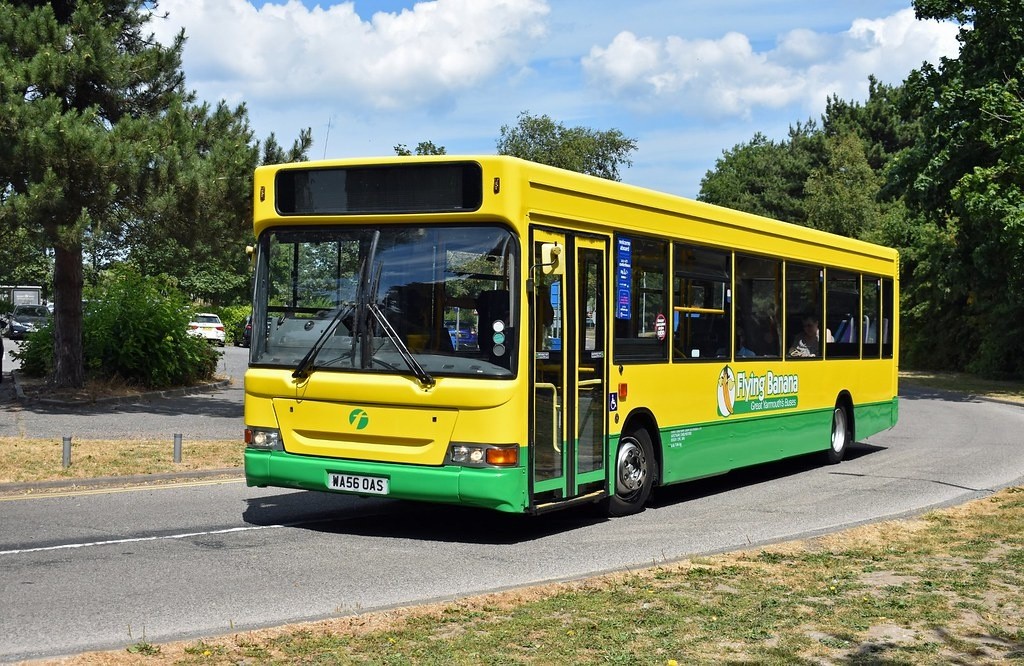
[716,329,755,357]
[315,287,382,336]
[793,315,835,355]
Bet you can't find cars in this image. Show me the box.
[186,313,227,347]
[82,300,109,317]
[46,303,54,314]
[10,304,54,340]
[234,315,286,349]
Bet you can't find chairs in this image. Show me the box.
[828,312,889,343]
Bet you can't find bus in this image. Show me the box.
[242,154,900,517]
